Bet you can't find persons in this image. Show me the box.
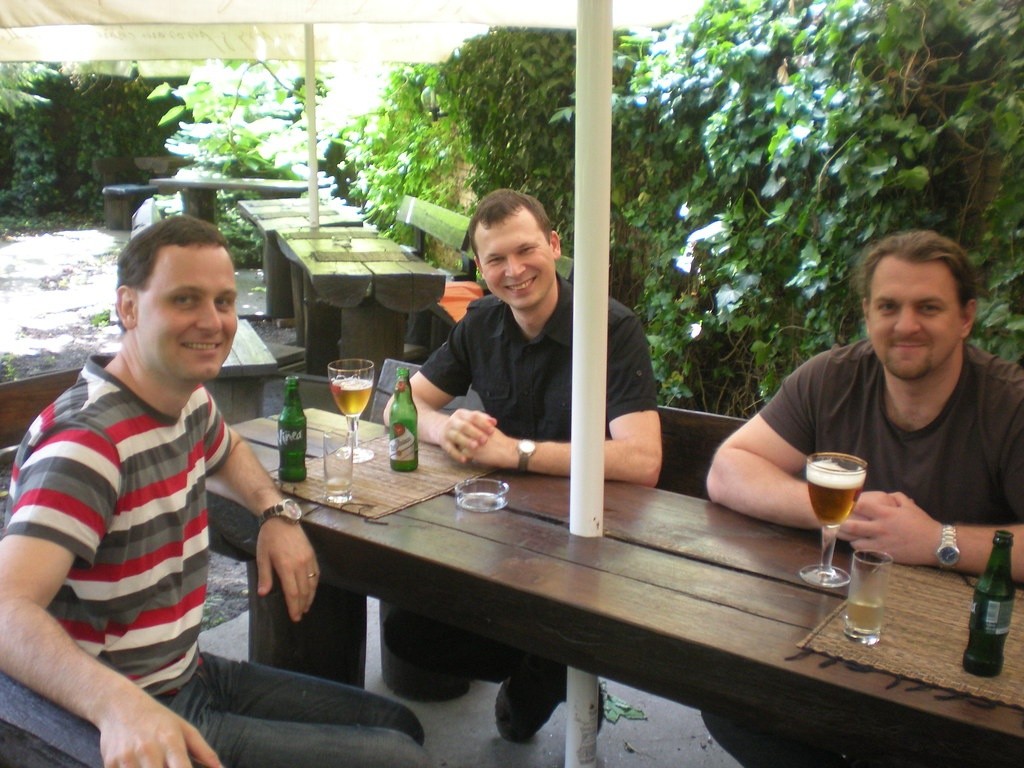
[0,214,429,768]
[701,233,1024,768]
[383,188,661,743]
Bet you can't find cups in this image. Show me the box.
[322,429,355,504]
[842,551,894,646]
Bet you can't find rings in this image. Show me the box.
[308,574,315,577]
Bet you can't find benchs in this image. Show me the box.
[394,194,471,359]
[102,184,154,230]
[429,234,575,355]
[210,316,277,425]
[94,155,150,186]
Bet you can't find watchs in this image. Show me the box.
[257,498,302,528]
[517,438,535,472]
[937,525,960,570]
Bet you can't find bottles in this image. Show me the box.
[962,530,1017,678]
[277,376,307,483]
[388,365,418,472]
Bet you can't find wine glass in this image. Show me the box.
[799,452,868,589]
[327,359,375,464]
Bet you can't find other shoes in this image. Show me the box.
[493,677,562,742]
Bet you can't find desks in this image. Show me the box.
[133,154,194,178]
[149,175,447,385]
[205,407,1024,768]
